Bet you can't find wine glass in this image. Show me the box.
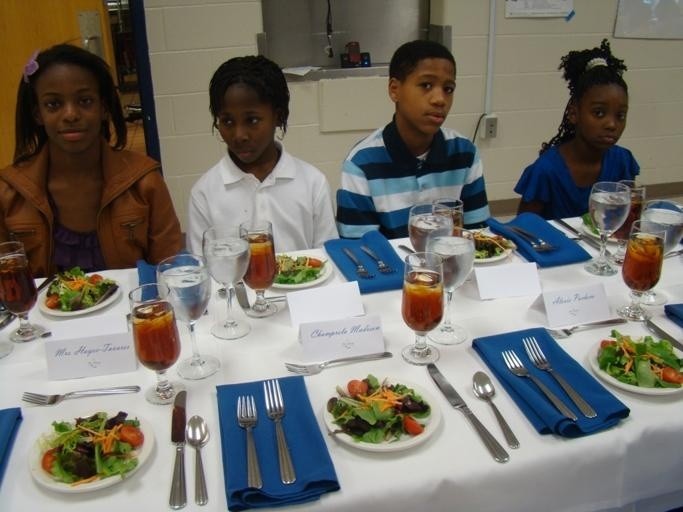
[401,197,476,366]
[583,179,683,323]
[0,241,46,343]
[127,219,277,405]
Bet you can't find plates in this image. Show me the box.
[38,278,121,316]
[462,228,512,263]
[28,415,155,494]
[581,221,619,242]
[588,334,683,395]
[272,252,332,289]
[323,375,441,453]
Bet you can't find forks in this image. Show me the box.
[22,385,140,407]
[237,379,297,489]
[341,244,393,278]
[509,226,559,254]
[285,352,393,375]
[500,336,597,426]
[545,318,627,339]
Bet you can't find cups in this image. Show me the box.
[331,31,354,69]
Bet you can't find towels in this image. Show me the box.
[603,200,683,245]
[216,375,341,512]
[485,211,594,269]
[324,228,415,295]
[472,327,630,439]
[664,303,683,327]
[0,406,24,491]
[136,248,208,316]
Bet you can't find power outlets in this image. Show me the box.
[476,115,499,139]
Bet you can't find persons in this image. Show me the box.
[0,43,185,279]
[513,38,640,221]
[335,38,492,241]
[185,54,340,258]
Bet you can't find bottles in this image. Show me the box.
[340,42,371,67]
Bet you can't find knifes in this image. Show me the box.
[644,319,683,351]
[168,391,187,510]
[554,218,624,266]
[234,279,250,313]
[427,364,510,463]
[0,270,57,329]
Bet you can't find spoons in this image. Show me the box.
[185,415,211,506]
[472,371,519,449]
